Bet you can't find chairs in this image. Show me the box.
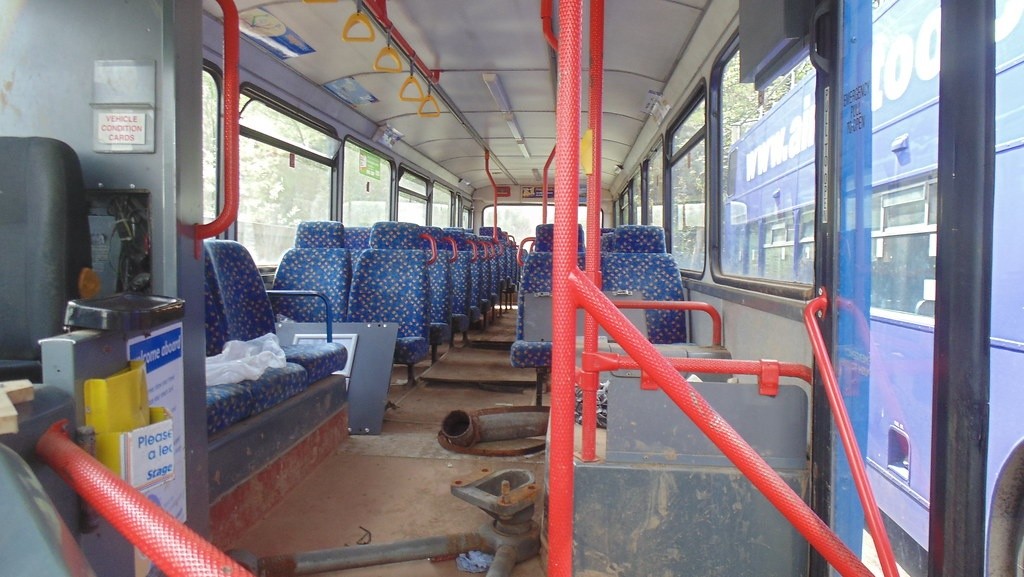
[204,220,687,437]
[0,136,93,380]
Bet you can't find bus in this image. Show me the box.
[720,1,1024,577]
